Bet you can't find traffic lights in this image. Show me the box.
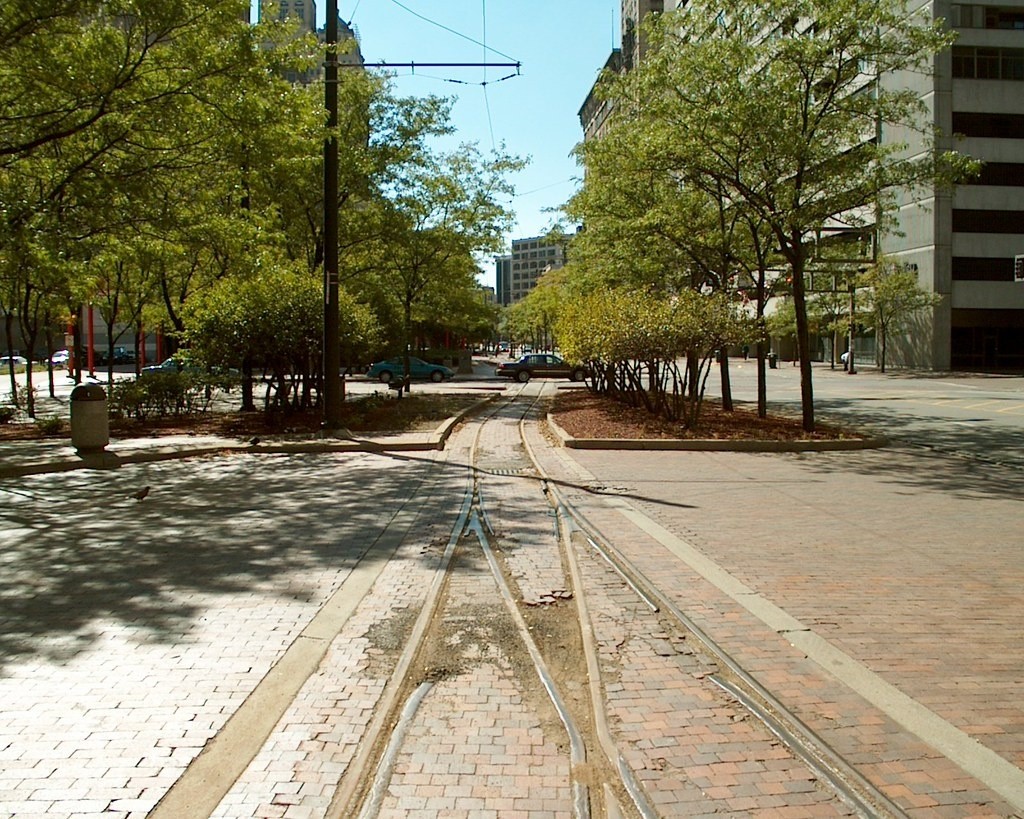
[1015,254,1024,281]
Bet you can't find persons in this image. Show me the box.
[841,352,850,371]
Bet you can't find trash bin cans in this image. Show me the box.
[443,355,453,369]
[69,382,110,453]
[715,350,721,362]
[767,353,777,369]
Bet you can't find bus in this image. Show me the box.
[499,342,509,354]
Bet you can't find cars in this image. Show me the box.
[0,344,104,367]
[101,347,128,365]
[125,351,138,364]
[367,356,456,383]
[524,344,533,355]
[495,354,592,383]
[140,353,240,382]
